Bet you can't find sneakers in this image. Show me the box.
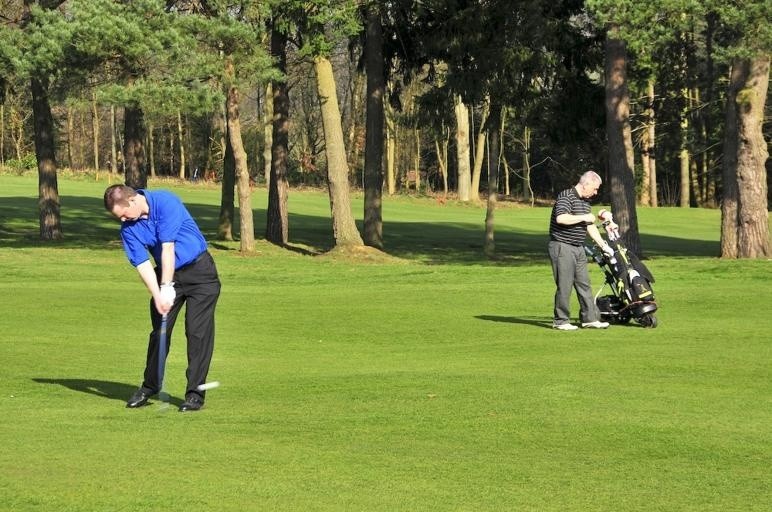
[552,324,578,330]
[582,320,609,328]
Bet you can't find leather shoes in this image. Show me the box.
[127,388,160,408]
[180,395,203,411]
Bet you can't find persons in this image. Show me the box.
[103,183,221,411]
[547,171,614,331]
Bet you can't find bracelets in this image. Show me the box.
[159,282,176,287]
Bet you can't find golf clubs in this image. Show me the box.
[156,312,168,384]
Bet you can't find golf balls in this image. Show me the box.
[197,380,220,393]
